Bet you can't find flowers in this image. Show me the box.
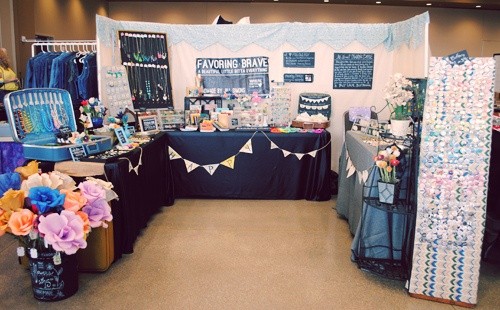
[0,161,117,260]
[386,74,413,121]
[79,97,107,123]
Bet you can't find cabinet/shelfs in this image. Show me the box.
[356,90,423,280]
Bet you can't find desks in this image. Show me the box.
[55,128,331,262]
[333,129,412,267]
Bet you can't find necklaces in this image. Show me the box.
[41,102,54,131]
[49,103,61,129]
[19,108,45,134]
[13,109,27,138]
[122,35,166,63]
[125,66,171,105]
[59,104,70,124]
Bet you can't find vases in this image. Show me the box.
[92,118,103,126]
[390,120,411,136]
[24,247,79,301]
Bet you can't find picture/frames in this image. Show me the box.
[138,115,158,132]
[114,127,129,145]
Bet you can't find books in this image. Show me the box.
[199,123,217,132]
[213,121,230,132]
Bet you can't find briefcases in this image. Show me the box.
[4,87,111,161]
[291,92,332,128]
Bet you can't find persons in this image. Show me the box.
[0,47,22,90]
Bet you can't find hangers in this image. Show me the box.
[39,41,97,63]
